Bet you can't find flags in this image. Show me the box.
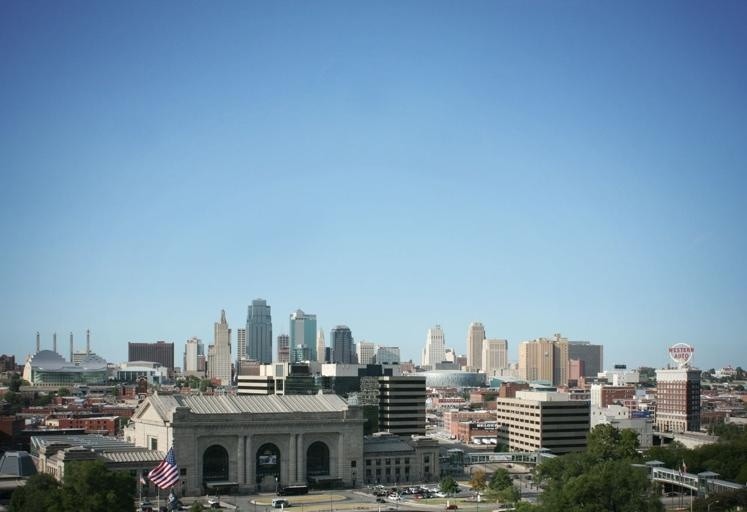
[140,477,147,486]
[147,446,179,490]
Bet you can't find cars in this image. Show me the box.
[208,499,216,504]
[369,483,458,511]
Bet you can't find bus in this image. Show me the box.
[271,499,289,508]
[277,485,308,496]
